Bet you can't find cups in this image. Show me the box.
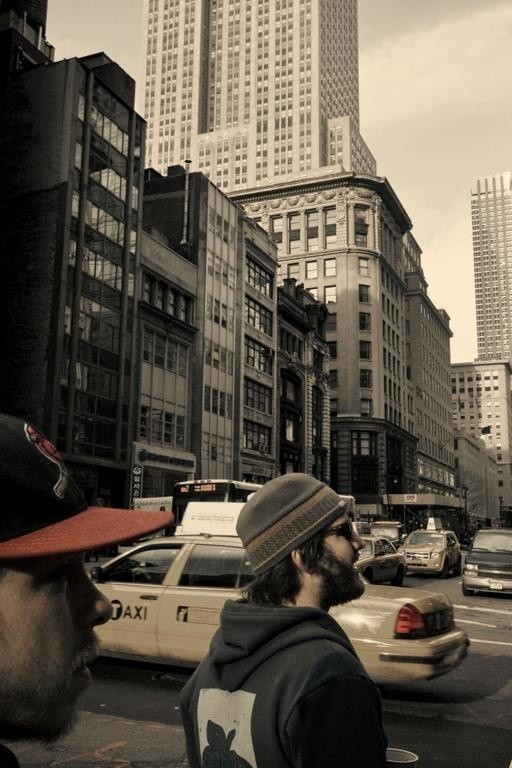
[387,747,419,767]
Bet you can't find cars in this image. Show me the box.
[460,528,512,597]
[397,517,463,578]
[95,500,472,690]
[348,537,409,585]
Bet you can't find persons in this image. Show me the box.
[1,414,178,766]
[180,474,390,768]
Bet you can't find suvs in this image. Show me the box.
[369,521,409,548]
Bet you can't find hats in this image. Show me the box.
[237,474,345,575]
[0,414,173,559]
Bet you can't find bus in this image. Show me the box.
[172,481,356,535]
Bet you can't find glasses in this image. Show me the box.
[326,520,354,540]
